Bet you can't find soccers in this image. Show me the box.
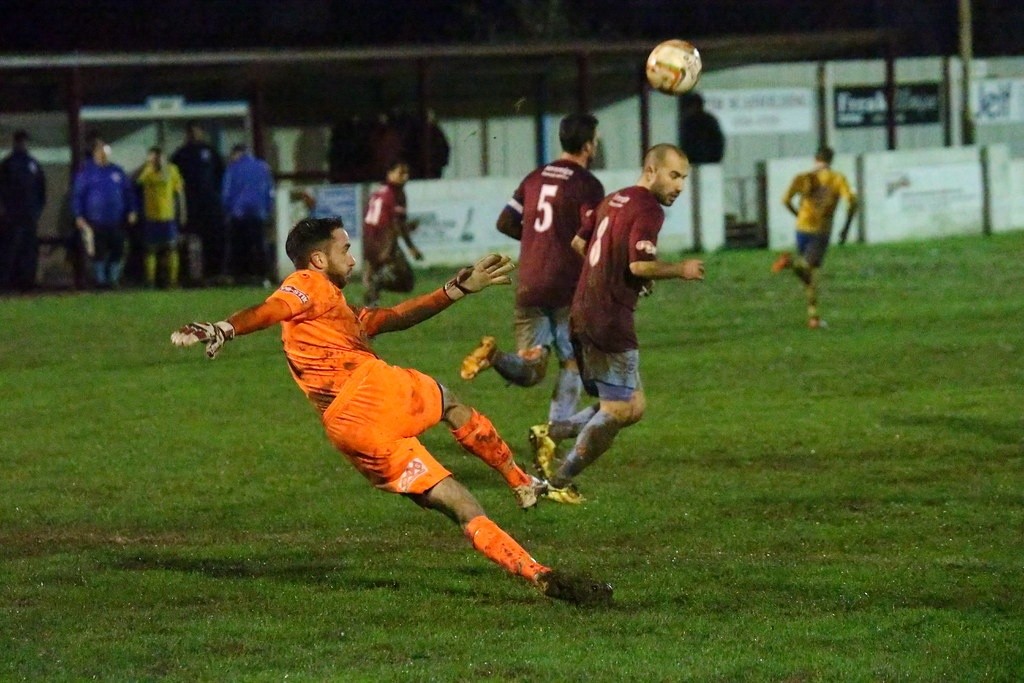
[646,38,703,97]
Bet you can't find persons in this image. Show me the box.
[0,131,47,294]
[364,107,448,179]
[679,93,723,164]
[219,144,273,288]
[460,114,605,463]
[169,121,223,285]
[60,133,201,289]
[171,216,612,603]
[361,161,423,310]
[773,145,859,327]
[530,144,706,503]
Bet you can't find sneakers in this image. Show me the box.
[538,568,612,601]
[513,473,550,510]
[530,422,558,481]
[544,478,587,505]
[460,336,497,379]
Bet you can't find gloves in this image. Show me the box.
[444,253,515,302]
[170,320,235,358]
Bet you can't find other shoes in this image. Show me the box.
[810,319,828,328]
[772,253,791,271]
